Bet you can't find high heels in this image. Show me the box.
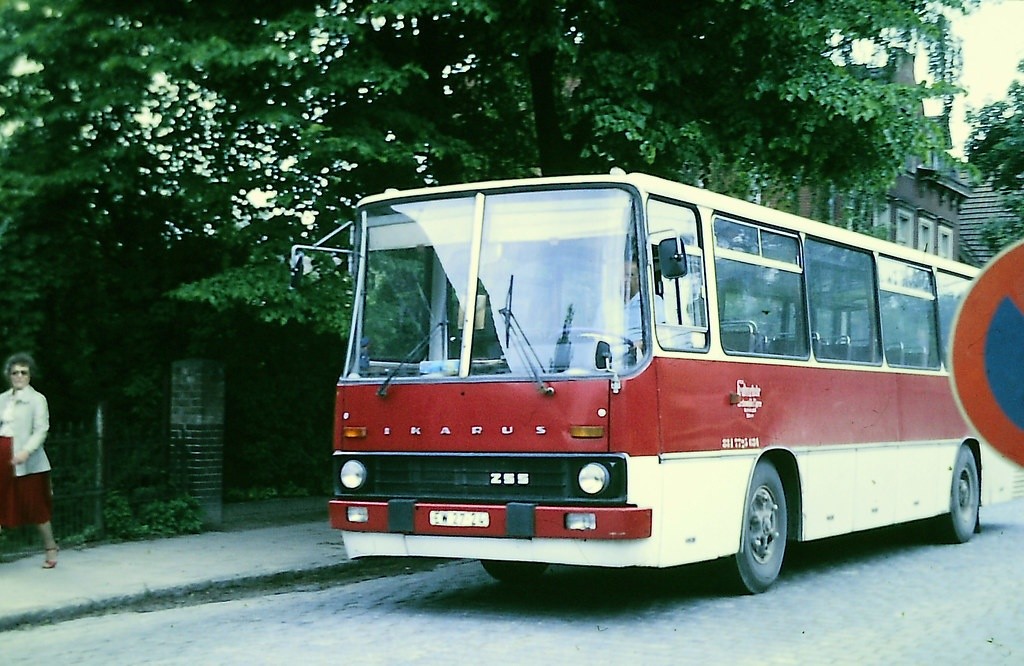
[42,546,59,568]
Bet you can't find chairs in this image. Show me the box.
[693,307,950,375]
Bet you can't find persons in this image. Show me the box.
[1,353,63,569]
[596,256,684,360]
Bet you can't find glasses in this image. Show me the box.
[10,369,29,376]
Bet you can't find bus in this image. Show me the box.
[286,170,1024,597]
